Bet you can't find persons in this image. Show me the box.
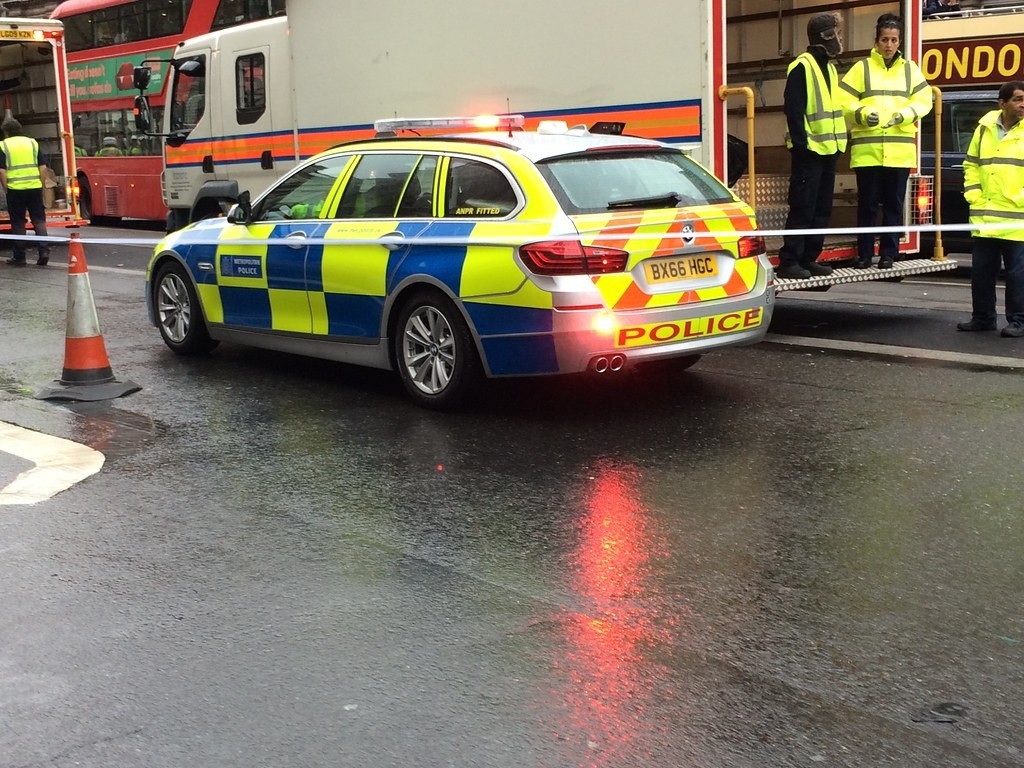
[95,135,124,156]
[927,0,950,19]
[290,162,367,218]
[0,118,52,266]
[776,12,847,279]
[957,80,1024,336]
[122,135,153,155]
[922,0,938,20]
[837,13,933,269]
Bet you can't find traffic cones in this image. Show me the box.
[32,235,141,401]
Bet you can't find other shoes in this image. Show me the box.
[776,265,811,278]
[1000,321,1024,337]
[7,258,26,265]
[957,319,997,333]
[800,262,833,275]
[36,252,49,265]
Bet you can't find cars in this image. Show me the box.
[920,90,1001,248]
[144,112,775,407]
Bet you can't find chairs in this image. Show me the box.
[364,170,422,217]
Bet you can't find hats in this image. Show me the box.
[806,12,843,57]
[1,118,22,133]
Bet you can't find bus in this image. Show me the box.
[919,0,1024,130]
[49,0,287,225]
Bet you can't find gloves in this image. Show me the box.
[880,114,904,129]
[867,112,879,127]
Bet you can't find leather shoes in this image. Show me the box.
[854,255,872,269]
[877,256,892,269]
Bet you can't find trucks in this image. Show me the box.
[131,0,961,300]
[1,18,90,232]
